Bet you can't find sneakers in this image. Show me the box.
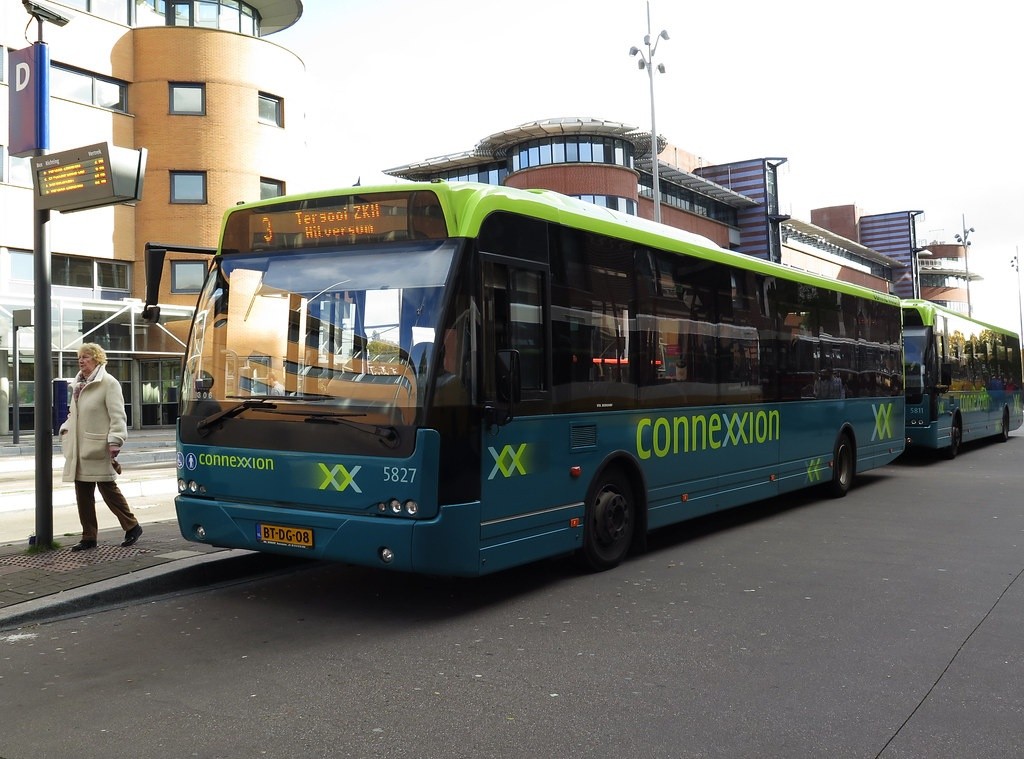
[121,525,143,547]
[71,539,97,551]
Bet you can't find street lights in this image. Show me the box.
[631,1,671,228]
[1011,245,1024,385]
[954,212,974,316]
[17,0,68,551]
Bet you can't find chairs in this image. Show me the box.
[407,342,461,406]
[545,339,877,410]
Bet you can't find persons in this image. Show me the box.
[58,342,142,551]
[987,375,1017,400]
[266,372,285,396]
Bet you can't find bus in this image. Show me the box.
[899,299,1024,459]
[142,181,911,578]
